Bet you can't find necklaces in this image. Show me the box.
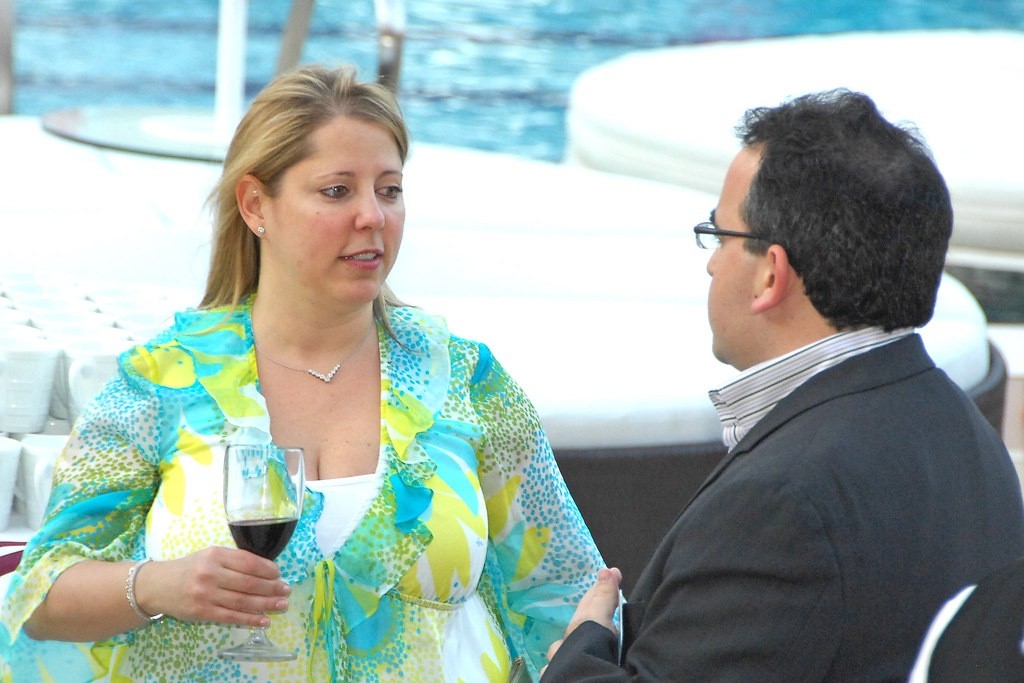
[253,319,380,382]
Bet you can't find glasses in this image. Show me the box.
[693,221,802,278]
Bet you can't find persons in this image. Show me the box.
[538,86,1023,683]
[0,66,628,682]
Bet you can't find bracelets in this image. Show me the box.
[125,557,163,622]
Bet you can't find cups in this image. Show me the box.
[0,342,122,535]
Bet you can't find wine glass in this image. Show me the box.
[218,445,305,660]
